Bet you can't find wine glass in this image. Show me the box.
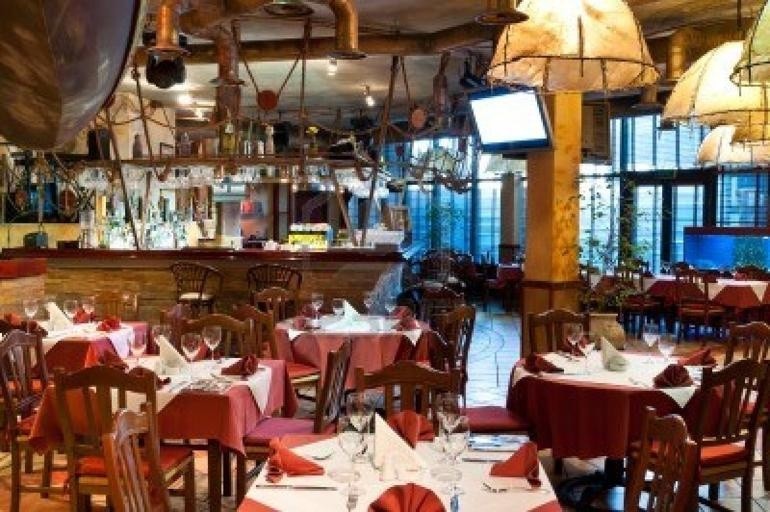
[658,335,676,369]
[129,322,223,379]
[336,391,471,510]
[312,292,397,334]
[651,260,674,280]
[563,324,583,366]
[23,294,95,340]
[642,324,659,365]
[578,333,595,376]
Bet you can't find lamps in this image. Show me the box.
[327,57,337,76]
[365,85,375,106]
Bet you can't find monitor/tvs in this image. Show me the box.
[459,83,555,156]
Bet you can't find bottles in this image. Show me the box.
[81,196,189,251]
[348,129,356,151]
[221,107,277,158]
[133,132,143,158]
[179,132,191,156]
[308,130,319,155]
